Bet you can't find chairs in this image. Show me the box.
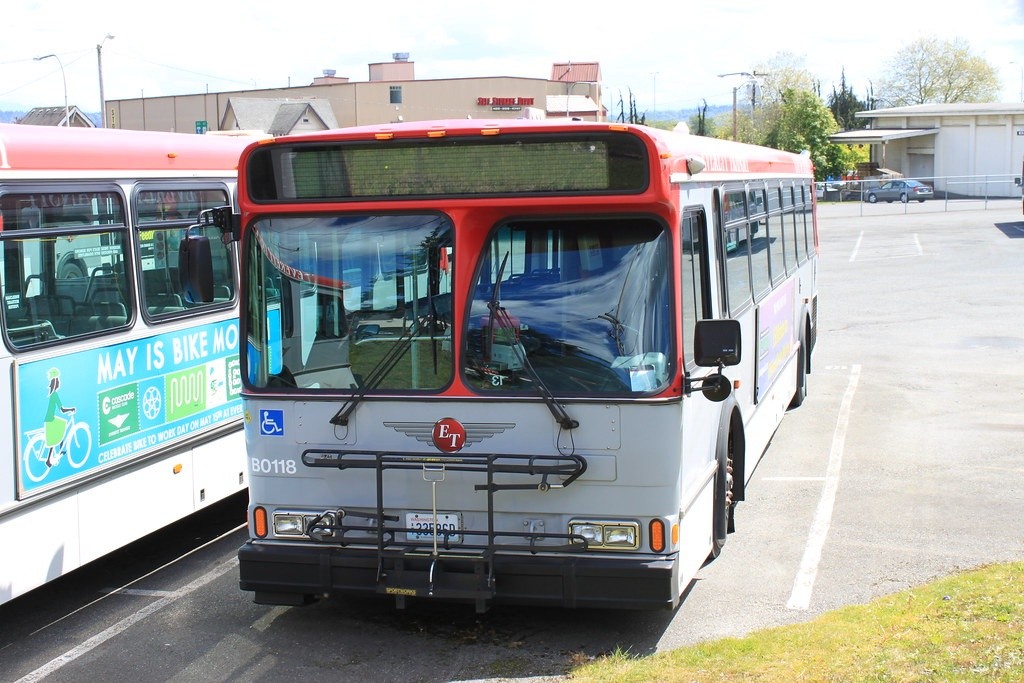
[350,267,644,370]
[6,264,231,347]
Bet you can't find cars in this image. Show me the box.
[864,179,934,203]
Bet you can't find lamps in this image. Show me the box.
[1018,131,1024,135]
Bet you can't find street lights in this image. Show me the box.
[566,82,599,117]
[717,72,763,130]
[33,54,70,127]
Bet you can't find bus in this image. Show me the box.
[179,107,817,611]
[0,122,276,605]
